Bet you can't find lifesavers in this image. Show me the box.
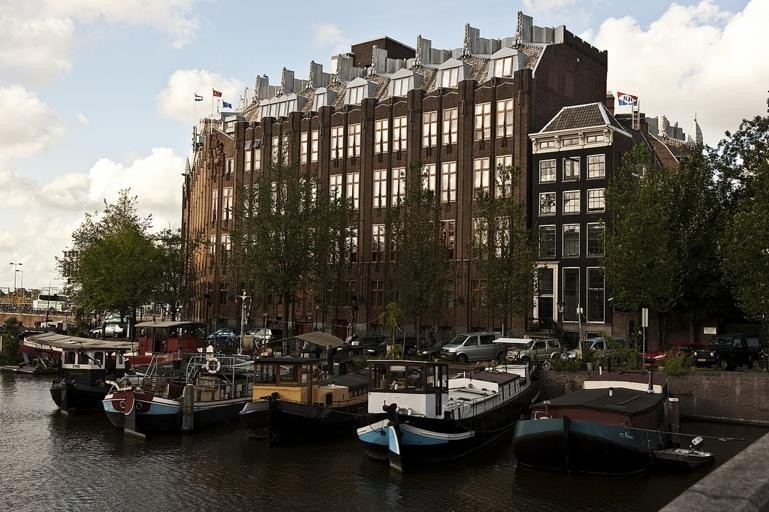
[206,357,221,374]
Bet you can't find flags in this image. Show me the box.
[223,102,232,108]
[213,90,223,97]
[617,91,639,106]
[195,94,203,101]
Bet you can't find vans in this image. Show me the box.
[439,330,506,364]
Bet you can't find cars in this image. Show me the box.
[344,335,635,369]
[637,340,704,372]
[90,310,275,353]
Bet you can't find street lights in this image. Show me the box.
[576,302,585,349]
[556,297,565,334]
[234,290,253,354]
[16,269,24,312]
[8,260,23,311]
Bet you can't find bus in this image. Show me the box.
[37,292,72,315]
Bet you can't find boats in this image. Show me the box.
[106,320,209,369]
[102,353,256,430]
[240,330,373,431]
[0,360,39,374]
[509,369,674,487]
[352,355,544,453]
[49,341,131,414]
[654,448,717,467]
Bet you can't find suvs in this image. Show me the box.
[691,332,763,370]
